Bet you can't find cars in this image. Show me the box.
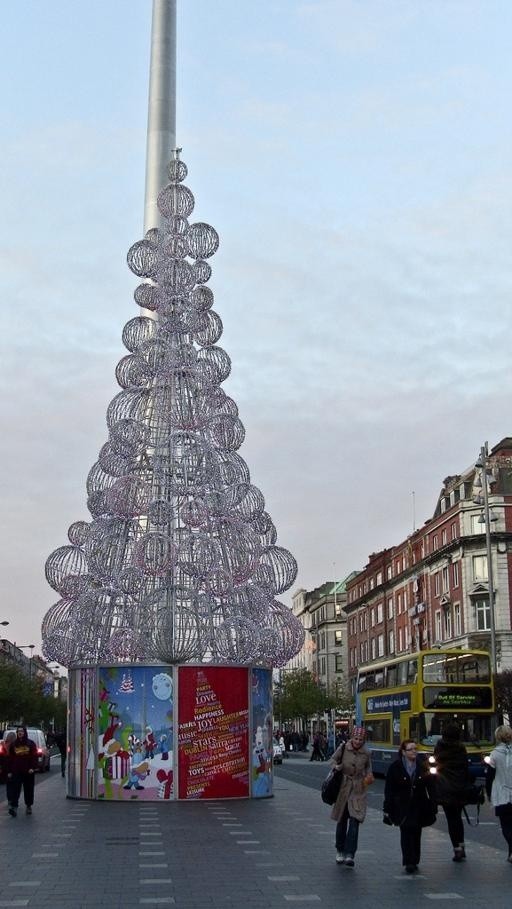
[272,735,283,764]
[0,727,55,776]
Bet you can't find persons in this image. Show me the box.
[434,725,470,862]
[384,739,436,874]
[328,726,376,868]
[5,725,39,816]
[56,727,67,776]
[274,727,348,761]
[484,725,512,863]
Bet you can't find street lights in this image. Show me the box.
[0,620,9,628]
[43,665,60,676]
[473,438,498,693]
[13,642,36,672]
[278,666,298,741]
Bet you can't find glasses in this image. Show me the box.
[404,747,417,752]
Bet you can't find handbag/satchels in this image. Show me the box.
[319,765,346,806]
[459,782,485,807]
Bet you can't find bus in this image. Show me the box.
[353,649,495,785]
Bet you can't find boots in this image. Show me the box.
[452,847,463,862]
[458,842,466,859]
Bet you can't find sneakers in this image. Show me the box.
[404,863,419,874]
[335,847,347,865]
[345,851,355,867]
[26,807,33,815]
[8,806,19,818]
